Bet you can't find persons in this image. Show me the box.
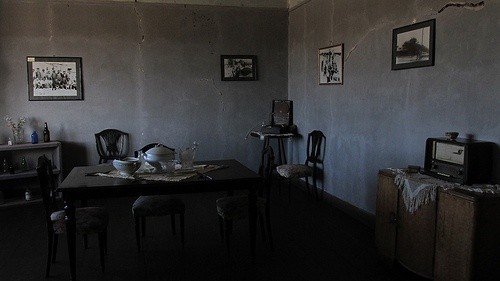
[32,67,77,90]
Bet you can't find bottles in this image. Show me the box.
[262,121,266,127]
[8,138,13,145]
[43,122,50,142]
[181,148,193,170]
[31,130,38,144]
[3,160,14,174]
[192,142,200,161]
[21,159,26,170]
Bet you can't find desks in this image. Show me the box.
[55,158,262,281]
[251,131,301,176]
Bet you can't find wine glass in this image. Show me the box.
[159,161,177,177]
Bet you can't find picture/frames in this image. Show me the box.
[391,18,436,70]
[317,43,344,84]
[26,56,84,100]
[220,55,257,80]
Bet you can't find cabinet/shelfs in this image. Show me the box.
[375,167,500,281]
[0,140,63,207]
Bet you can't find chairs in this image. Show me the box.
[276,130,326,215]
[215,147,275,267]
[37,155,110,281]
[131,143,186,262]
[95,129,129,165]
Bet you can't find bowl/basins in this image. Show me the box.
[144,144,176,170]
[113,157,142,176]
[446,132,459,141]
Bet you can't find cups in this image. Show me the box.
[26,192,31,200]
